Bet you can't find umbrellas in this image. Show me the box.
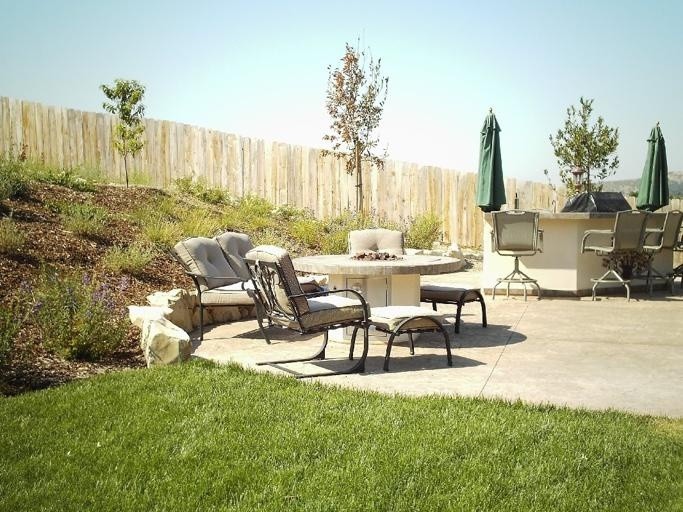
[635,120,670,212]
[474,106,507,213]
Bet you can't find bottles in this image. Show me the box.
[514,192,520,213]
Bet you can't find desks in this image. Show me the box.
[291,255,463,344]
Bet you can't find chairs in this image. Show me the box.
[347,229,405,255]
[491,209,542,301]
[171,235,329,345]
[216,229,330,288]
[242,244,371,379]
[580,209,683,301]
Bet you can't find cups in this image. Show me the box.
[407,249,414,265]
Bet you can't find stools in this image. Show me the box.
[348,305,454,372]
[420,281,488,335]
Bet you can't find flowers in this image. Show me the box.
[348,249,404,262]
